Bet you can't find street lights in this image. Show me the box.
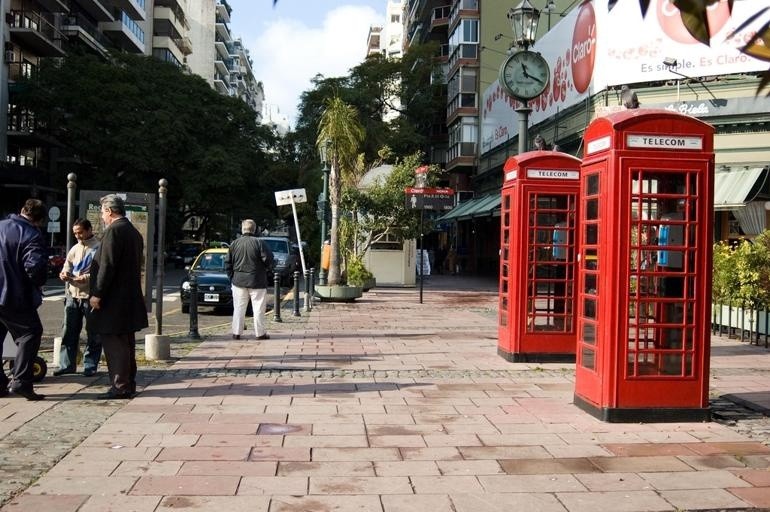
[506,1,542,156]
[312,133,334,287]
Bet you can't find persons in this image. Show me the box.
[54,216,101,377]
[1,198,54,401]
[224,218,276,341]
[85,193,150,400]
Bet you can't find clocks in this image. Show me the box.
[498,50,551,104]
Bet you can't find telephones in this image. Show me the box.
[651,237,658,262]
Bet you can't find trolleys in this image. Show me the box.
[1,331,48,382]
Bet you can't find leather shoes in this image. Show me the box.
[96,390,135,400]
[0,379,46,401]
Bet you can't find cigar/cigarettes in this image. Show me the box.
[90,304,97,313]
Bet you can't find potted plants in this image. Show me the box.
[339,141,449,294]
[314,96,364,303]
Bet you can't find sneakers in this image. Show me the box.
[256,334,270,340]
[233,334,240,339]
[52,367,76,376]
[83,366,97,376]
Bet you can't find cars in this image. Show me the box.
[45,245,66,275]
[170,232,311,317]
